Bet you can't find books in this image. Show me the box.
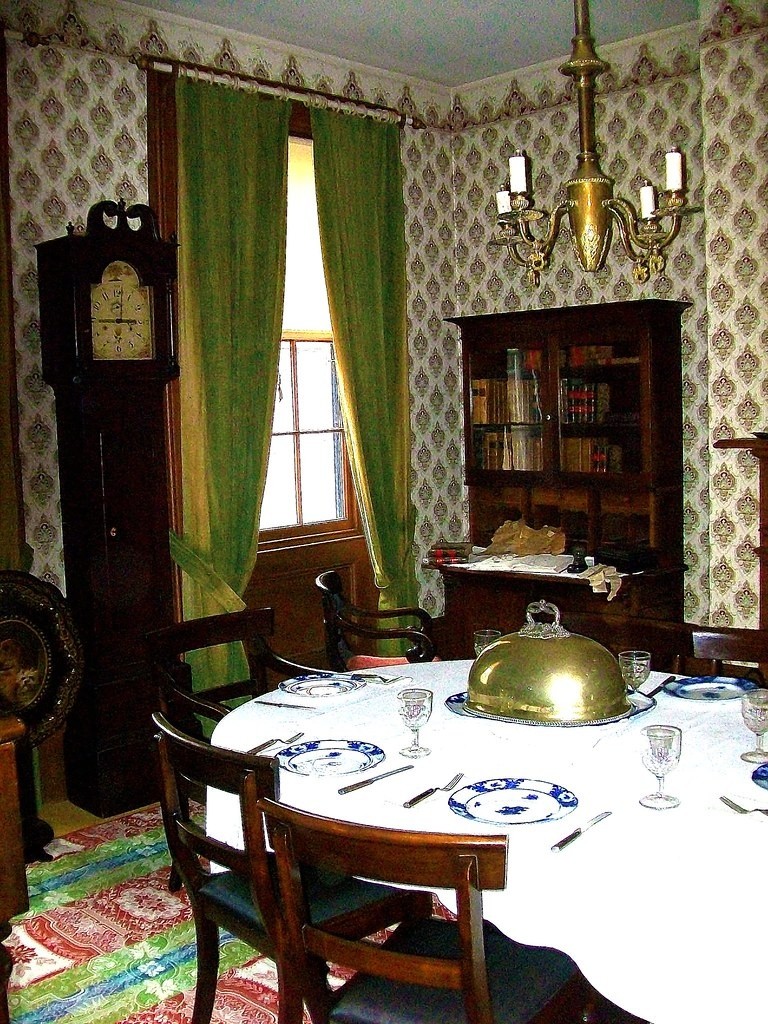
[558,343,638,473]
[467,554,573,574]
[470,379,542,472]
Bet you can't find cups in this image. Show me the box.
[473,629,501,657]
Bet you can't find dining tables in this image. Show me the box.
[205,661,768,1024]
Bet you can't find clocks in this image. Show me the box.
[35,198,182,813]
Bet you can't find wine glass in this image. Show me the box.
[639,725,682,810]
[617,651,651,710]
[396,688,433,758]
[741,689,768,763]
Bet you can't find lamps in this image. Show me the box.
[493,0,701,288]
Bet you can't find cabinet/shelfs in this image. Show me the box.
[436,298,693,675]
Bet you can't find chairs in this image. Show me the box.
[150,709,431,1024]
[256,797,598,1023]
[315,568,442,673]
[144,605,333,892]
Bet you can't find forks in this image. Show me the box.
[718,795,768,817]
[404,773,465,808]
[353,674,403,683]
[249,731,302,755]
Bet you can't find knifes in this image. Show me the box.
[552,812,612,852]
[647,676,676,697]
[254,700,316,709]
[339,763,415,795]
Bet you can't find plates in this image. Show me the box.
[277,673,365,699]
[275,739,386,777]
[448,778,578,827]
[752,763,768,788]
[663,675,756,702]
